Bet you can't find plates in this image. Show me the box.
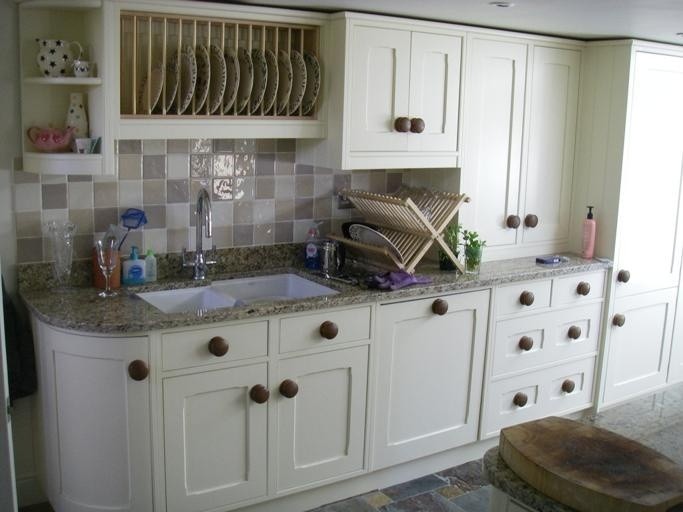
[346,223,404,265]
[139,43,322,118]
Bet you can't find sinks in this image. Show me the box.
[128,280,240,313]
[208,267,343,307]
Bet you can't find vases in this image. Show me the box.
[37,41,84,78]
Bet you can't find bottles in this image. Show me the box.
[143,249,158,283]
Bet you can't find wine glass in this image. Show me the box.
[42,219,119,299]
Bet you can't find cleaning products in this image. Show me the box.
[581,204,597,259]
[144,249,157,282]
[123,245,146,285]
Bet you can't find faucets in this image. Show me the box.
[182,187,218,279]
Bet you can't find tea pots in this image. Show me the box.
[25,124,76,153]
[32,36,82,77]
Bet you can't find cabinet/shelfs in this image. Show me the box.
[107,0,330,143]
[581,36,683,415]
[16,0,107,176]
[457,24,584,263]
[296,7,462,171]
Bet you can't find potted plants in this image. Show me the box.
[461,230,488,274]
[438,223,463,270]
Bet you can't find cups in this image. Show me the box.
[320,239,346,277]
[74,136,101,154]
[73,59,92,78]
